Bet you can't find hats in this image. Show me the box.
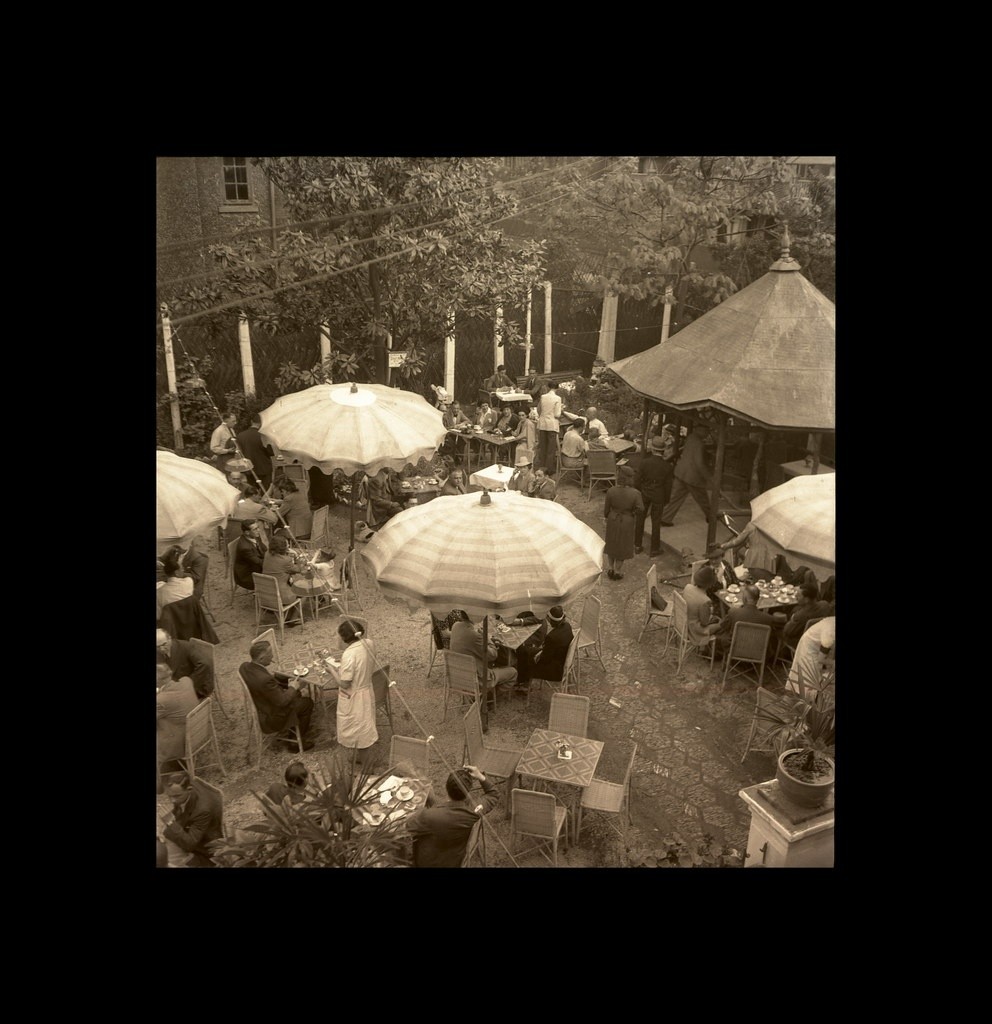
[649,419,709,451]
[694,567,718,587]
[702,542,725,560]
[515,456,532,469]
[618,466,633,478]
[547,606,565,621]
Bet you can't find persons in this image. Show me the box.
[156,544,220,774]
[440,364,609,500]
[239,621,378,765]
[210,413,342,626]
[368,467,406,522]
[733,419,783,507]
[433,606,574,700]
[604,424,720,579]
[407,765,499,867]
[683,521,835,698]
[263,762,308,816]
[157,771,224,867]
[307,466,337,509]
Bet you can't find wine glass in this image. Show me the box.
[369,804,381,823]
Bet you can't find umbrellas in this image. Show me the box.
[258,382,449,551]
[750,471,835,582]
[360,490,606,732]
[156,450,241,561]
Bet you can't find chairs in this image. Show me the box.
[192,776,228,868]
[461,815,487,867]
[157,696,226,794]
[389,734,430,778]
[510,784,570,867]
[462,700,522,819]
[157,376,835,766]
[574,744,639,852]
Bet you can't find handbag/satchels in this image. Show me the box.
[651,586,667,611]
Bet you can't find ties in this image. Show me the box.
[254,543,262,556]
[229,427,234,436]
[177,807,182,816]
[176,554,179,562]
[386,475,394,495]
[458,486,464,494]
[586,420,590,429]
[529,381,533,390]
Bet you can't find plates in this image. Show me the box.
[724,595,738,602]
[770,579,784,585]
[368,812,387,826]
[754,581,767,588]
[270,508,280,511]
[427,482,438,484]
[359,789,378,801]
[727,587,740,593]
[776,597,790,603]
[401,486,411,488]
[293,667,309,676]
[277,458,284,460]
[395,788,414,802]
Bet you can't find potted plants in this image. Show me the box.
[751,654,834,807]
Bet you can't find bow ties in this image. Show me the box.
[453,414,458,417]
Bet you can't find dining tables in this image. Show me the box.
[157,390,834,867]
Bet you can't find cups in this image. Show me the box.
[758,579,765,587]
[272,505,278,509]
[430,479,436,483]
[780,594,788,601]
[278,455,283,458]
[362,787,373,797]
[730,584,738,590]
[728,593,736,601]
[400,786,410,797]
[296,665,305,673]
[402,481,409,486]
[774,576,783,583]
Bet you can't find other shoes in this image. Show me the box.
[608,570,623,580]
[321,598,325,602]
[660,520,674,527]
[650,549,664,557]
[295,619,302,624]
[348,755,369,764]
[288,742,315,753]
[635,547,644,554]
